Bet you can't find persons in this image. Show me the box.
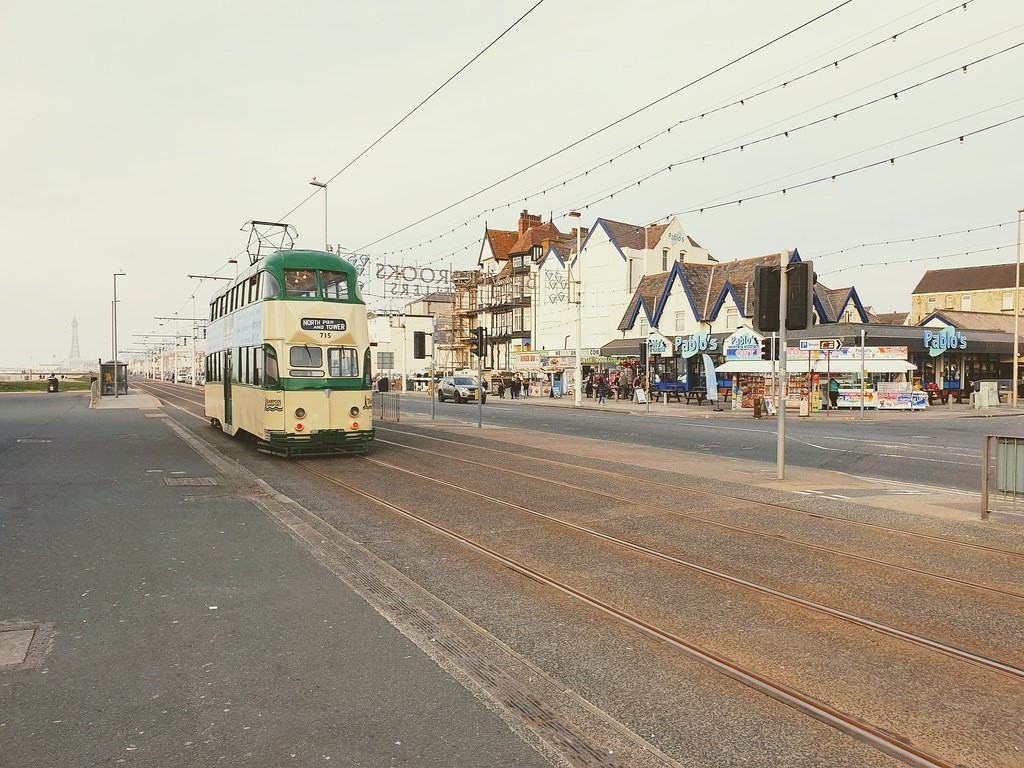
[825,378,840,410]
[482,379,488,390]
[596,378,609,406]
[509,377,530,399]
[498,382,505,399]
[583,369,643,396]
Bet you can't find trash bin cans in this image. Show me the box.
[48,378,58,393]
[800,397,808,416]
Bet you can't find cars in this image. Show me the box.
[150,371,205,386]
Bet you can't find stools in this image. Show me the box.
[587,385,732,405]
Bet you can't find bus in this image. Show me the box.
[202,247,376,460]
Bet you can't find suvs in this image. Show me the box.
[438,376,486,404]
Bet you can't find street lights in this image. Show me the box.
[567,212,584,408]
[111,299,121,360]
[311,180,328,253]
[113,272,127,399]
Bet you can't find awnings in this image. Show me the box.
[814,359,918,371]
[715,359,815,373]
